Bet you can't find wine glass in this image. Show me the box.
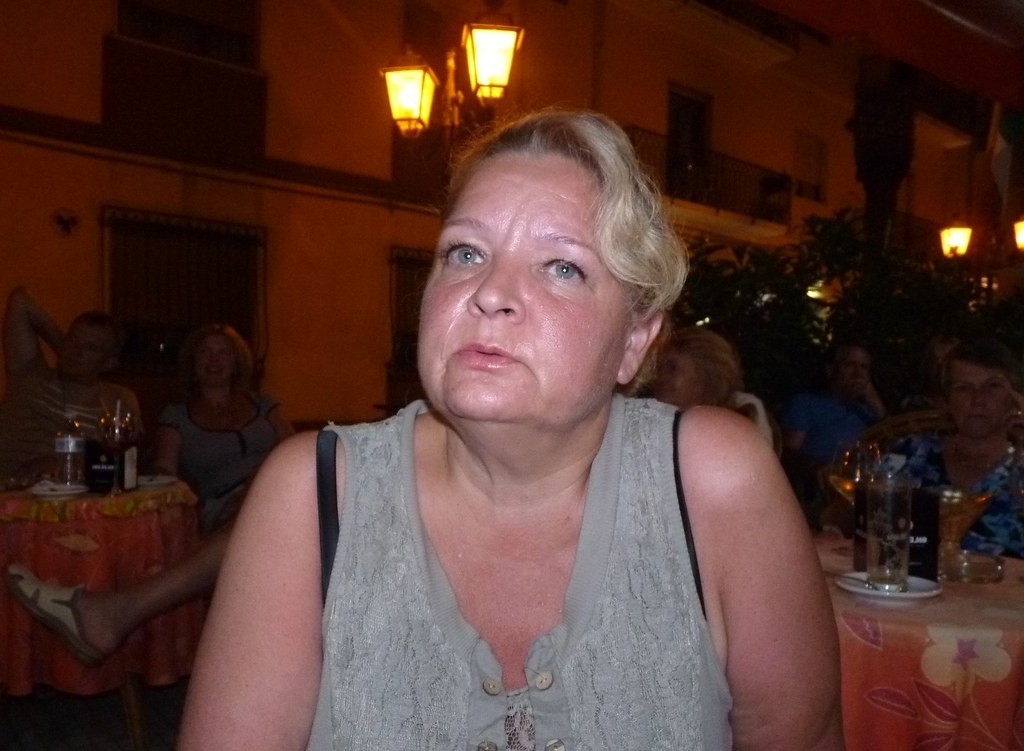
[828,442,881,558]
[949,549,1004,616]
[940,487,993,583]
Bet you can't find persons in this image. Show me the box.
[655,326,774,451]
[0,287,142,478]
[177,111,844,751]
[858,333,1024,558]
[153,322,297,534]
[0,519,235,662]
[782,331,888,468]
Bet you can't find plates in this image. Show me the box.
[834,571,942,605]
[27,486,88,500]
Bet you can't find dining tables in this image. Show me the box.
[813,537,1024,751]
[0,479,206,751]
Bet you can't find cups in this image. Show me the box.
[103,440,139,491]
[853,460,911,592]
[39,436,86,487]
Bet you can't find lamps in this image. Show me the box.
[461,18,525,100]
[378,50,441,138]
[940,221,973,258]
[1013,215,1024,250]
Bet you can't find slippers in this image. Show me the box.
[2,555,106,669]
